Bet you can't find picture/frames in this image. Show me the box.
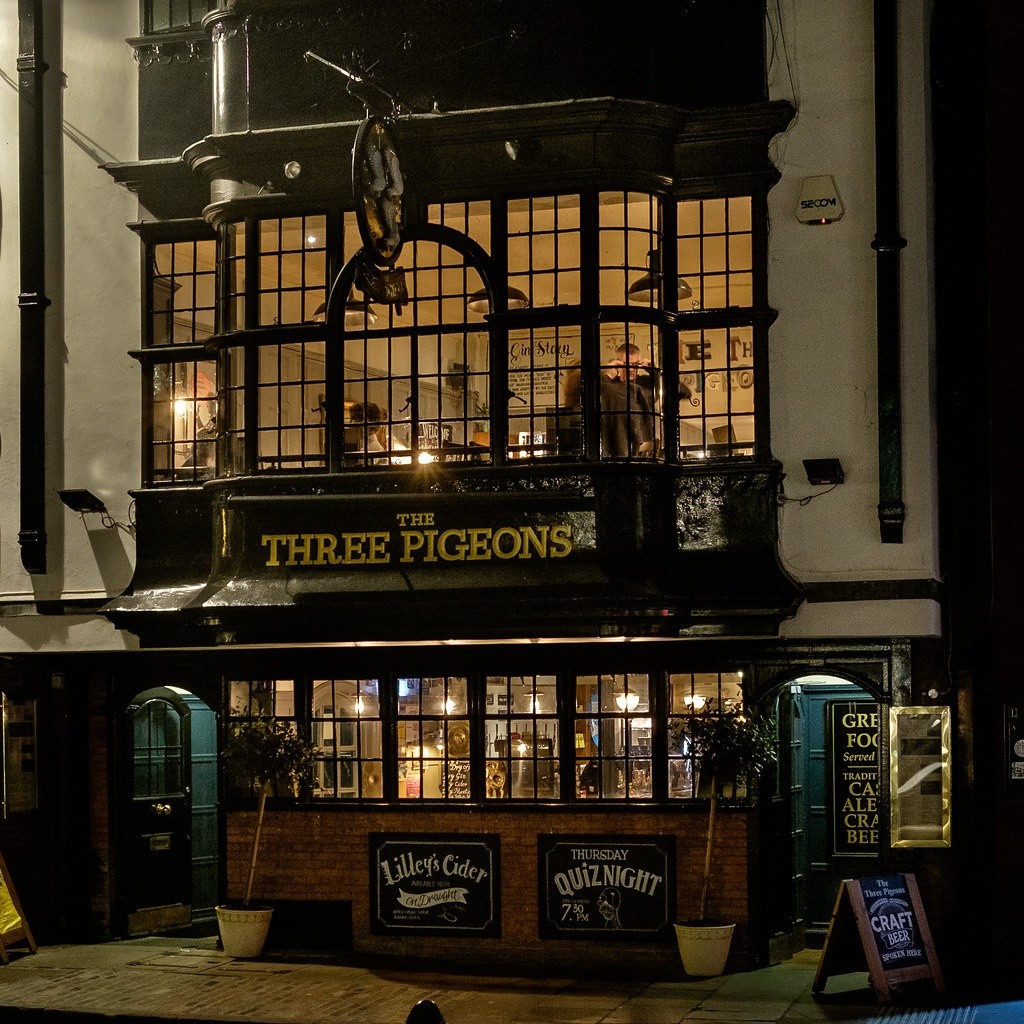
[888,706,952,848]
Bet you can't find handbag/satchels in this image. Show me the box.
[494,731,556,752]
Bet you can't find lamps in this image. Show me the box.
[504,133,534,162]
[613,687,636,695]
[523,686,545,696]
[627,198,692,302]
[280,161,301,179]
[467,286,530,315]
[311,286,379,329]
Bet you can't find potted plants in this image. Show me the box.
[214,704,327,958]
[666,696,780,977]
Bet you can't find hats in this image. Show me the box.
[349,402,381,419]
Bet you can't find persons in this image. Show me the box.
[601,343,694,459]
[567,361,643,458]
[576,760,599,795]
[343,401,389,468]
